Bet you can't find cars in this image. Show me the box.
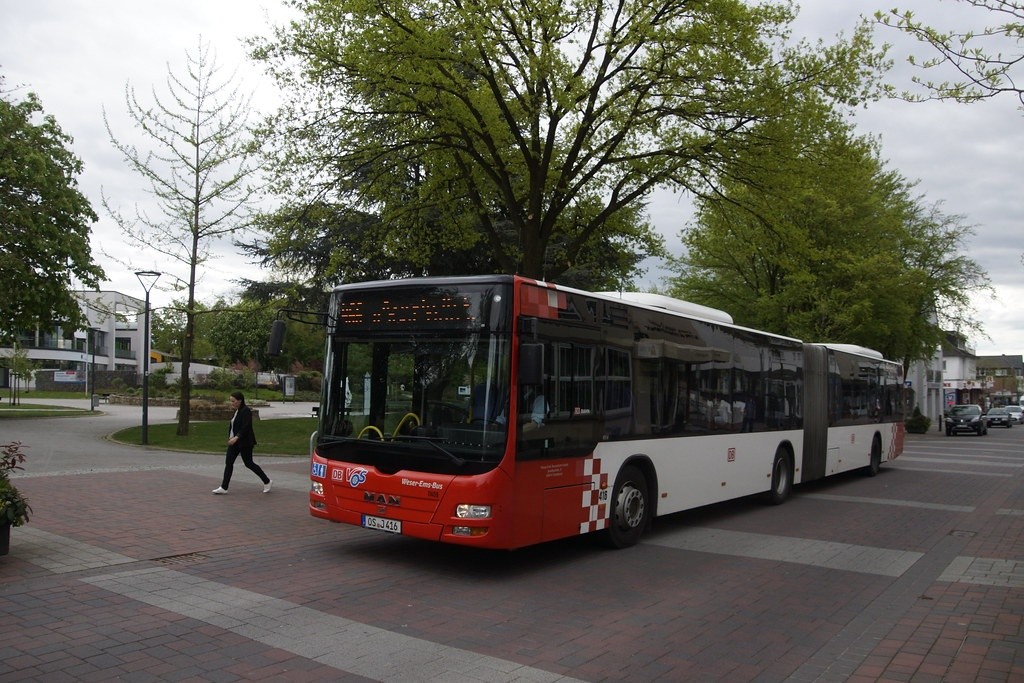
[987,408,1012,428]
[1005,406,1024,424]
[945,405,987,436]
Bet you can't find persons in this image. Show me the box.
[766,392,780,430]
[492,369,550,433]
[740,392,756,433]
[212,391,273,495]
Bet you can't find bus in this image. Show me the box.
[267,274,904,548]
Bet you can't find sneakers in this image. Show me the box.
[263,479,273,493]
[211,486,228,494]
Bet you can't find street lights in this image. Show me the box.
[89,327,101,414]
[136,272,161,445]
